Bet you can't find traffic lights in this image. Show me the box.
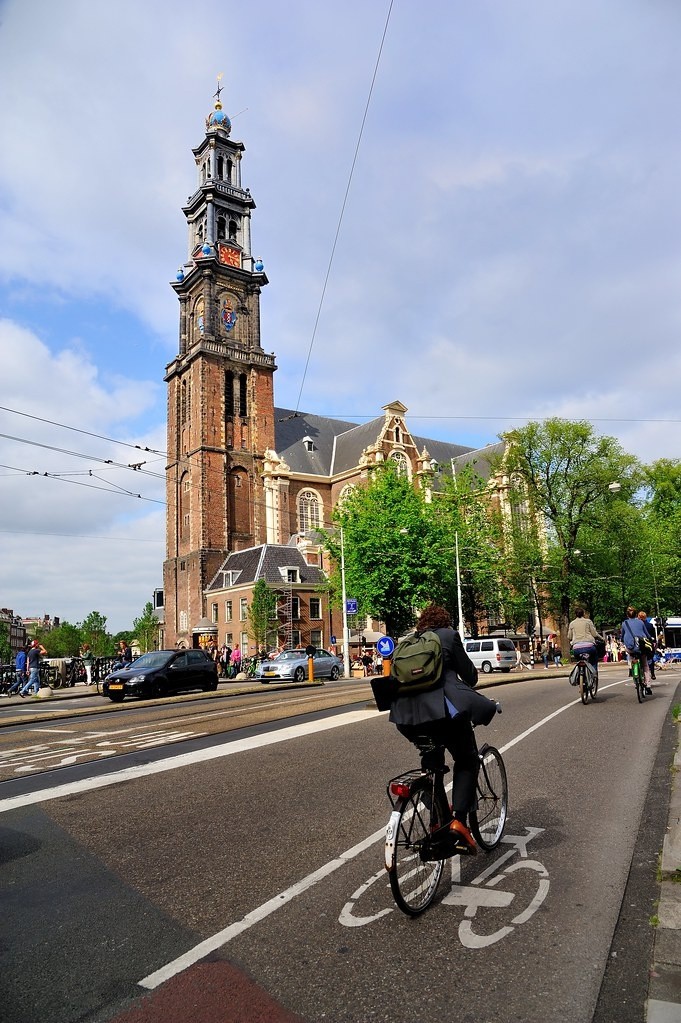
[524,622,529,635]
[530,625,536,635]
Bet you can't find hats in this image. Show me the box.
[30,640,39,647]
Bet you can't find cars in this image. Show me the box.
[102,649,219,704]
[254,648,344,684]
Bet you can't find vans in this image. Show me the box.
[461,638,518,673]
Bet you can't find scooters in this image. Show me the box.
[64,656,98,688]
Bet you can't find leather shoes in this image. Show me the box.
[428,804,452,832]
[449,819,477,856]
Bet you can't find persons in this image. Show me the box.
[7,639,48,699]
[388,605,497,856]
[207,639,241,679]
[605,638,627,662]
[515,647,523,672]
[112,640,133,672]
[535,642,563,669]
[80,643,93,687]
[621,606,656,695]
[567,608,606,678]
[360,650,383,677]
[250,644,268,670]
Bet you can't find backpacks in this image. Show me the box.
[391,627,443,694]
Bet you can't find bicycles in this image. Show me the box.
[97,660,117,696]
[3,660,63,695]
[623,639,653,704]
[578,639,606,705]
[653,650,681,672]
[383,699,510,919]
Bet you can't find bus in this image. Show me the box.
[635,617,681,662]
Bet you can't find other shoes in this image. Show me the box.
[646,687,652,695]
[18,693,26,698]
[544,667,548,669]
[651,676,656,680]
[629,668,633,677]
[8,690,12,698]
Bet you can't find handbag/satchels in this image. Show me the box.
[634,636,653,655]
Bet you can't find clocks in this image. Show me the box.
[218,243,240,268]
